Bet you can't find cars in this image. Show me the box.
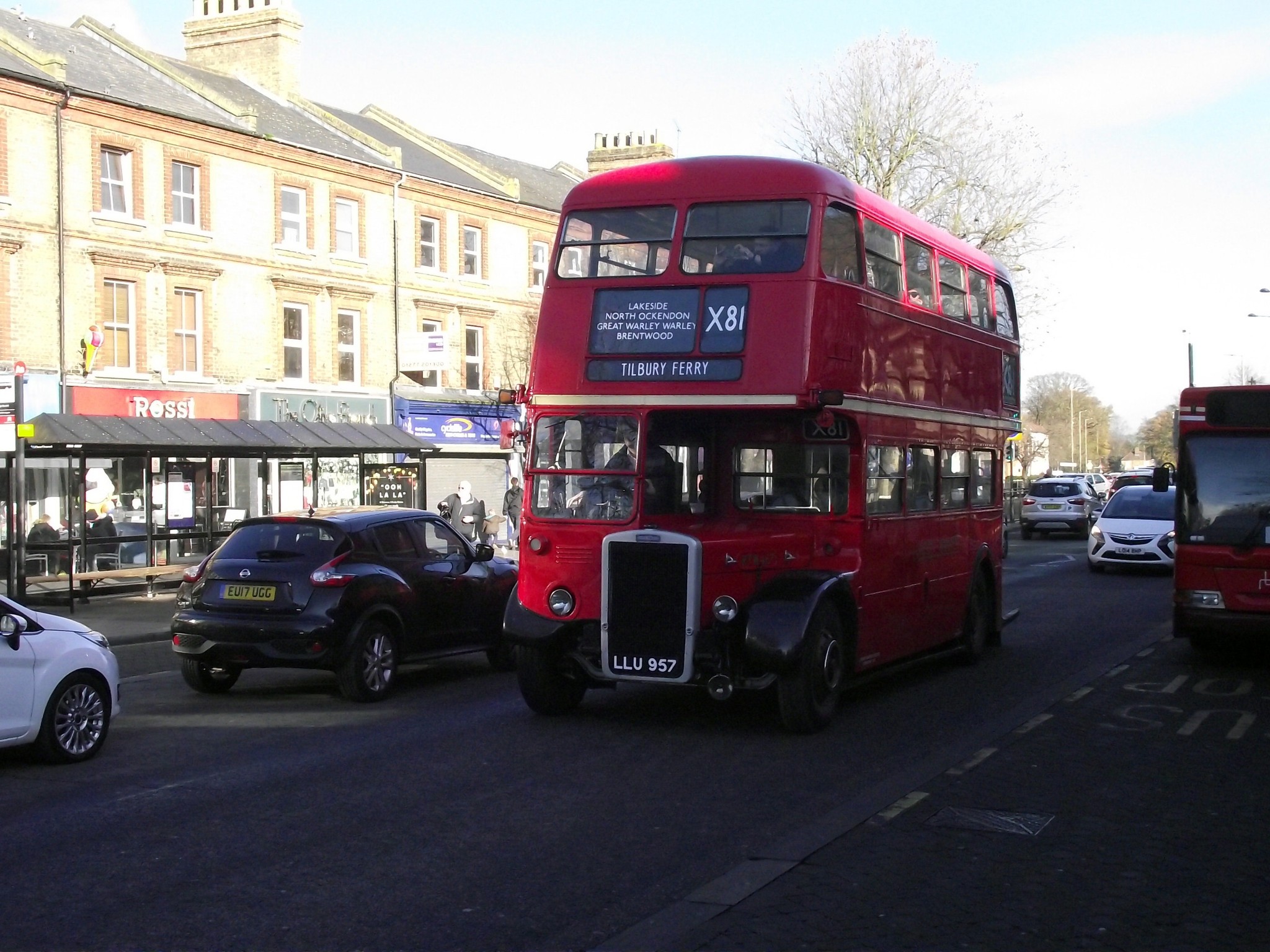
[169,503,522,704]
[1019,464,1177,501]
[0,594,122,767]
[1087,484,1176,575]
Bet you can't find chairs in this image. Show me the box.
[13,531,48,578]
[94,530,123,572]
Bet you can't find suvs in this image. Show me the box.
[1018,477,1107,541]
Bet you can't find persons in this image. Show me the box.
[438,481,479,554]
[482,509,507,548]
[564,429,676,516]
[502,477,524,549]
[78,509,117,572]
[28,514,62,575]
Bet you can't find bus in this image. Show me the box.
[496,152,1022,739]
[1152,380,1269,667]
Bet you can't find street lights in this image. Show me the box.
[1072,387,1093,473]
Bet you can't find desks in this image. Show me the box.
[55,536,80,575]
